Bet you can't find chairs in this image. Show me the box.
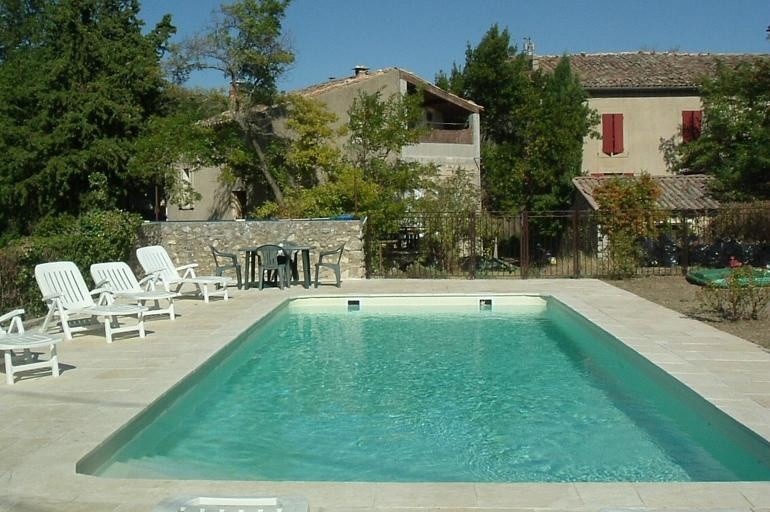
[1,245,231,386]
[207,240,347,291]
